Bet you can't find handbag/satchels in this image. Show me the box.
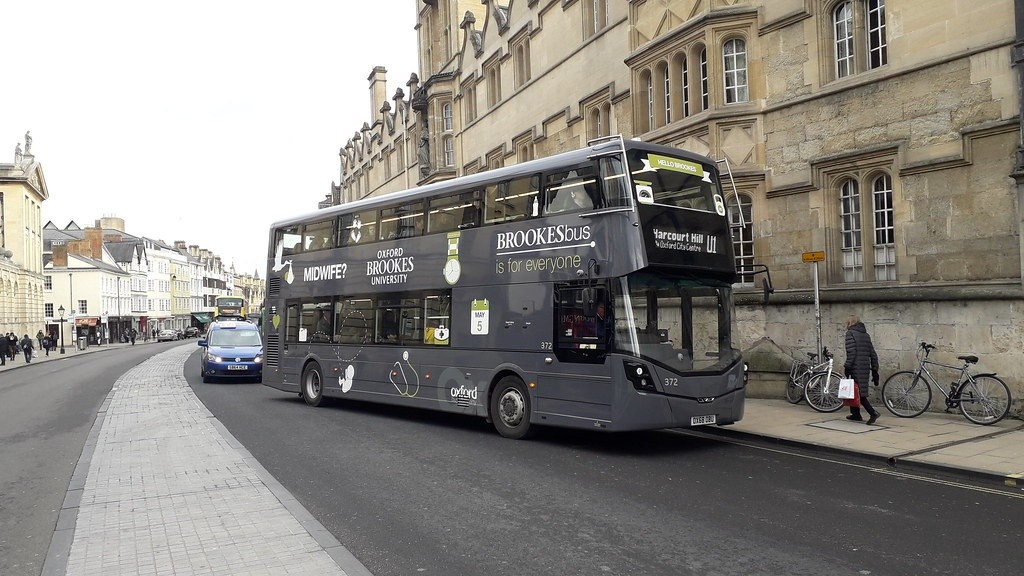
[23,344,29,351]
[838,377,854,399]
[843,383,860,407]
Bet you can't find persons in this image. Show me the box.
[36,330,44,350]
[124,327,129,343]
[51,331,58,351]
[42,333,52,356]
[363,225,375,242]
[406,217,424,236]
[843,314,880,425]
[152,329,156,338]
[310,235,324,249]
[130,329,137,345]
[317,305,330,343]
[20,334,34,363]
[0,332,18,366]
[382,311,399,338]
[417,121,428,165]
[581,301,604,342]
[25,130,33,154]
[15,143,23,156]
[309,307,322,335]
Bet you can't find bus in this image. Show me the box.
[214,296,249,321]
[248,313,262,334]
[261,134,775,439]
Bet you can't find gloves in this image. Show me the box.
[845,367,851,378]
[871,371,880,387]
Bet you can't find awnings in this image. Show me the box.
[193,314,212,323]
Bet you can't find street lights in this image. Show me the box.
[58,304,65,354]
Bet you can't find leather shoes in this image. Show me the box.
[867,411,880,425]
[846,414,862,421]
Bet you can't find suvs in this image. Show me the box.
[184,327,201,338]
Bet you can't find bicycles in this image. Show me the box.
[880,340,1011,426]
[785,346,847,412]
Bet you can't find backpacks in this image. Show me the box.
[43,339,48,346]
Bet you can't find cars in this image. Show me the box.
[216,314,245,322]
[157,330,184,342]
[197,321,264,384]
